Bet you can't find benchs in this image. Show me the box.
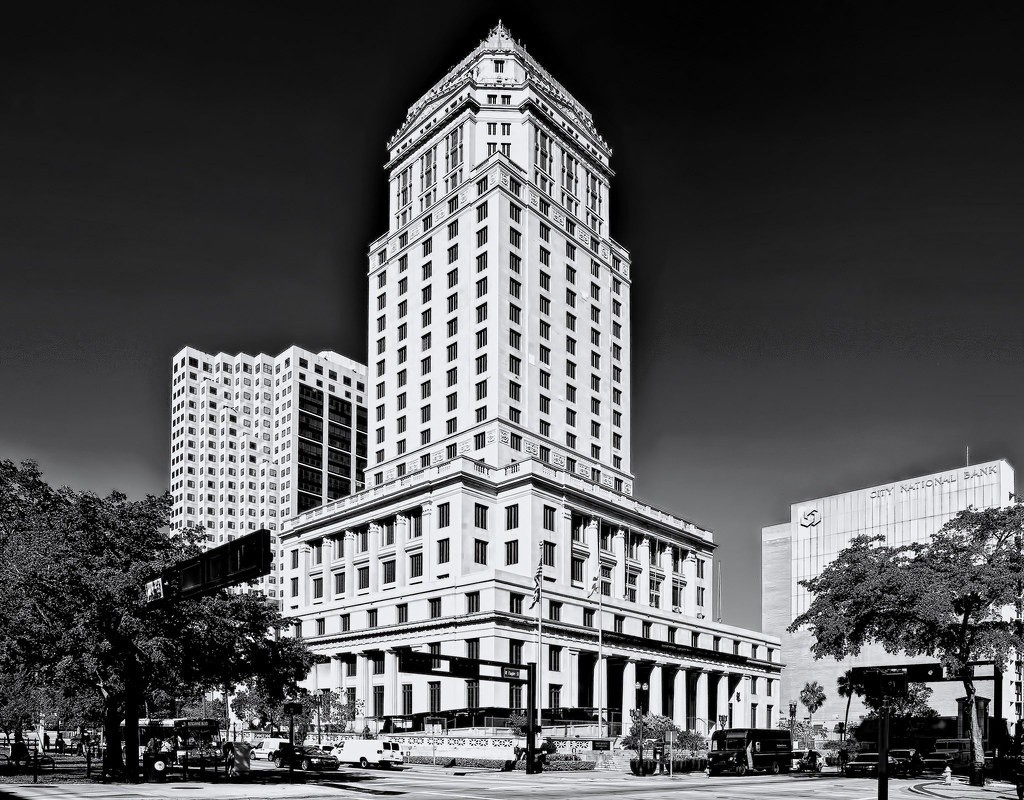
[10,742,55,770]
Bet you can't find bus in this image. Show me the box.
[120,718,224,765]
[707,728,793,776]
[934,739,970,766]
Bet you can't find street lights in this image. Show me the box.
[719,715,727,730]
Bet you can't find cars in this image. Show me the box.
[272,745,340,771]
[888,749,925,777]
[845,752,900,778]
[923,752,954,771]
[308,744,335,755]
[789,750,824,773]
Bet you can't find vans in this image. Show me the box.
[330,739,404,769]
[249,737,289,762]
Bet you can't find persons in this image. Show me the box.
[44,733,50,751]
[10,736,31,771]
[806,750,821,768]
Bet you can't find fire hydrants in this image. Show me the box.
[942,766,952,786]
